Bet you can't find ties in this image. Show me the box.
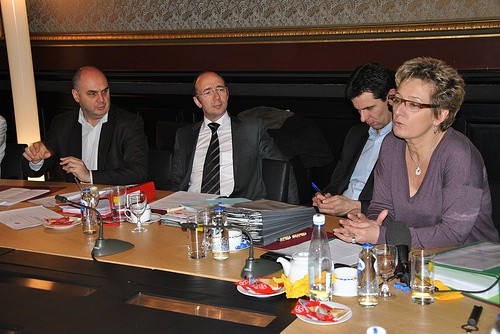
[201,123,220,195]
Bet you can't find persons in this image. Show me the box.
[312,62,397,217]
[168,70,299,205]
[332,56,500,249]
[20,65,150,185]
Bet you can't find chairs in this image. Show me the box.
[261,159,290,203]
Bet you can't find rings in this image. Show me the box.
[351,234,356,244]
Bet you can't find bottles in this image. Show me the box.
[211,206,229,261]
[307,215,334,303]
[80,189,97,235]
[357,243,379,308]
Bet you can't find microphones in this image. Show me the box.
[385,220,411,286]
[179,223,282,280]
[54,195,134,257]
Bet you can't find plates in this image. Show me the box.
[125,213,162,226]
[237,282,286,297]
[229,239,251,253]
[42,216,82,230]
[296,301,352,325]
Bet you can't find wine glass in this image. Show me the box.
[129,190,148,233]
[374,245,398,300]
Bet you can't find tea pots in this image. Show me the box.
[276,251,332,285]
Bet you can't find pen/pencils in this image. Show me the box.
[278,232,308,242]
[414,274,439,291]
[311,182,324,196]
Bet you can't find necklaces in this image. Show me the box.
[406,145,432,176]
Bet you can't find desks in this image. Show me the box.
[0,178,500,334]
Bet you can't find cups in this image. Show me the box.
[226,228,242,251]
[332,267,359,297]
[110,186,128,222]
[123,203,151,223]
[186,215,208,260]
[410,249,435,305]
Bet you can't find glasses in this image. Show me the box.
[387,94,440,113]
